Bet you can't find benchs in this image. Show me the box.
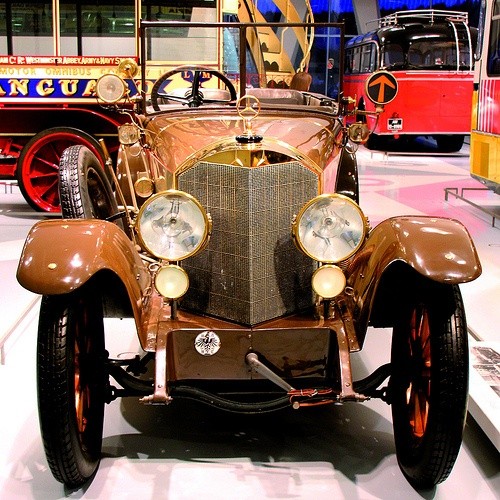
[146,88,330,106]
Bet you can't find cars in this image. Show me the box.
[17,19,483,490]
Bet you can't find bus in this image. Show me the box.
[343,10,474,151]
